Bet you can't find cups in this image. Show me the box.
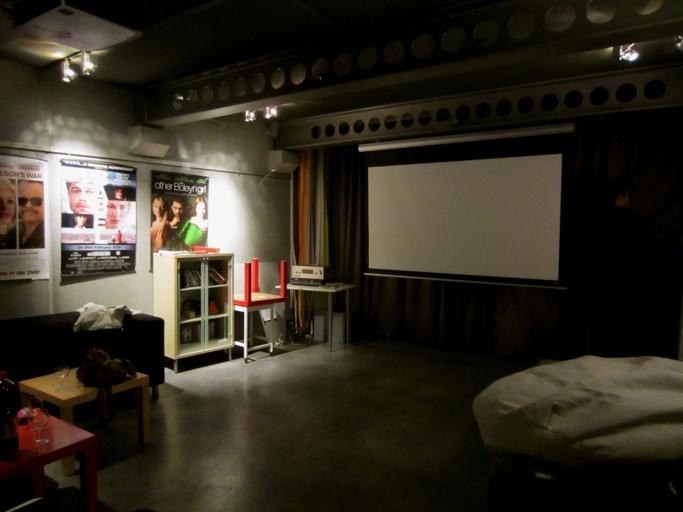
[177,221,204,248]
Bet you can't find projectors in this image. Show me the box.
[289,265,334,286]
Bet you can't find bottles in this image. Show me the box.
[0,372,20,459]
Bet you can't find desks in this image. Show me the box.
[0,409,99,511]
[234,257,287,364]
[18,367,152,477]
[274,281,359,352]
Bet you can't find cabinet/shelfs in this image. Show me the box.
[153,252,235,374]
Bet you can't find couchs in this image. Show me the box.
[0,307,163,421]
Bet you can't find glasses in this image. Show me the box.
[18,197,44,207]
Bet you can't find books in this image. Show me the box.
[181,267,227,343]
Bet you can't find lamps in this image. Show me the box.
[59,48,97,84]
[620,43,639,62]
[245,106,277,121]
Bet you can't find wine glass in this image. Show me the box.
[27,408,49,447]
[53,367,72,393]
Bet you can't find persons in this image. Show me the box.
[18,181,45,249]
[104,200,130,230]
[151,194,208,252]
[0,179,16,249]
[73,214,89,230]
[66,182,97,214]
[113,188,124,201]
